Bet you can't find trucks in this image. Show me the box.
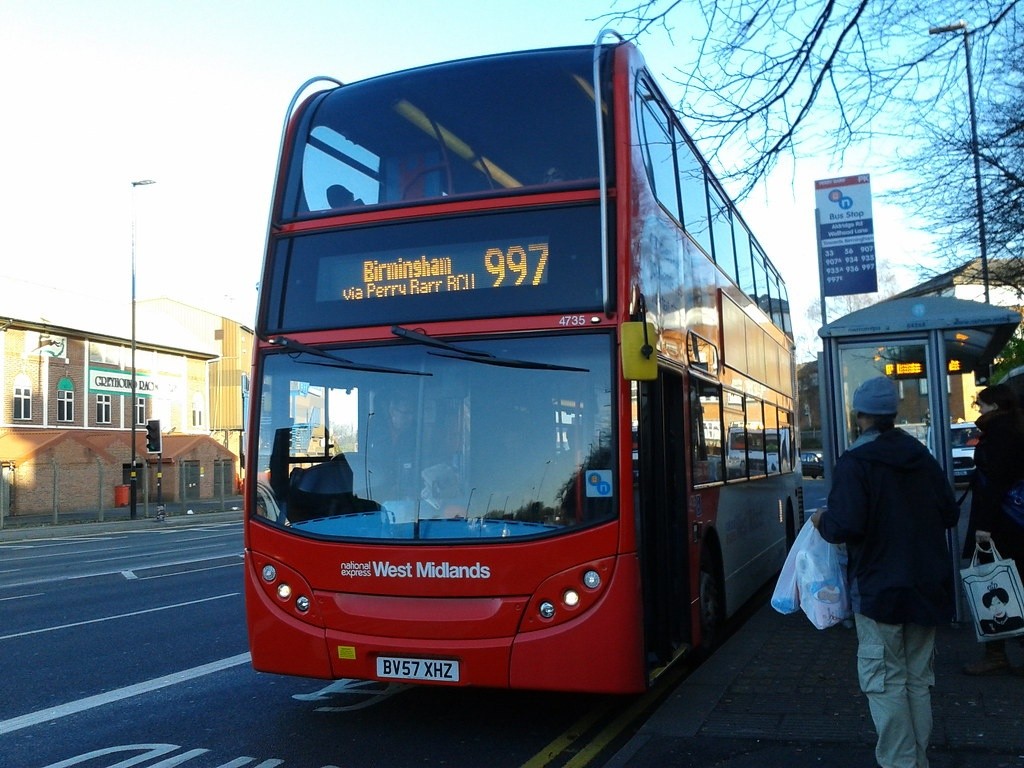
[727,426,790,477]
[928,422,982,485]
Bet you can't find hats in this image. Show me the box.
[853,376,898,416]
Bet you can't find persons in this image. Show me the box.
[810,377,963,768]
[960,382,1023,659]
[326,183,365,209]
[536,152,578,187]
[387,391,418,501]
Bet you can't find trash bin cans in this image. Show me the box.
[115,484,129,507]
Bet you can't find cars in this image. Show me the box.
[801,451,825,479]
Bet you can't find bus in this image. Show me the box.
[243,29,804,696]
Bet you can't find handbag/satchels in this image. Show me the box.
[979,476,1024,528]
[960,538,1024,643]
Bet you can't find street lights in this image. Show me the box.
[928,21,992,305]
[129,179,159,520]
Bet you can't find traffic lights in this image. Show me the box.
[146,419,162,453]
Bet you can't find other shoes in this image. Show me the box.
[963,650,1009,675]
